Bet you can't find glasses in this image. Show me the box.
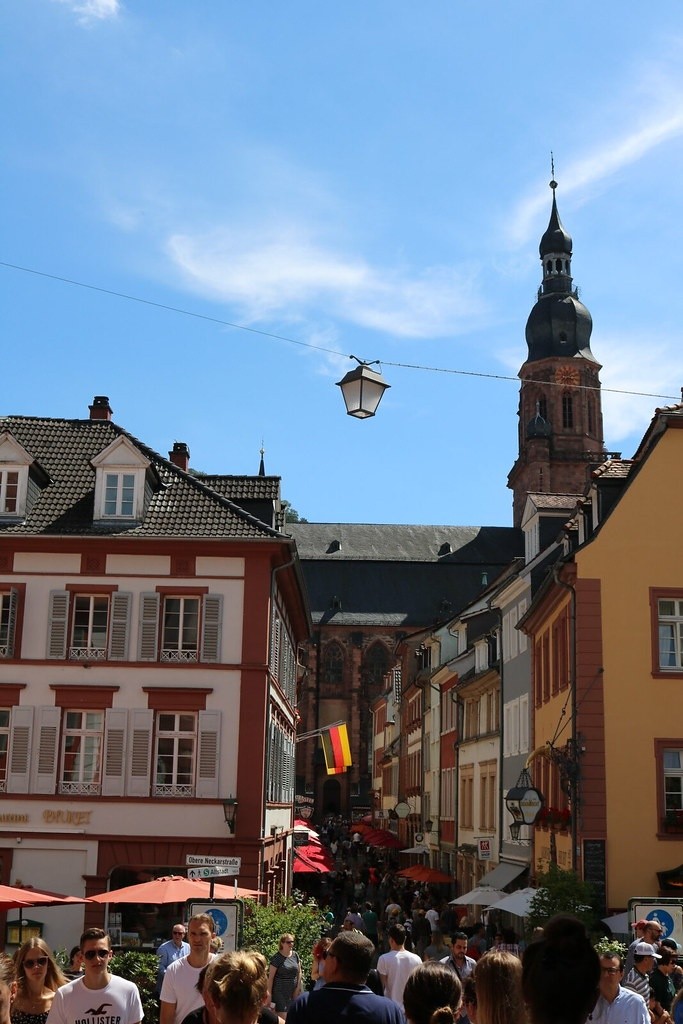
[84,950,110,960]
[670,959,678,965]
[649,929,662,932]
[286,941,295,944]
[635,928,638,930]
[173,931,183,935]
[602,967,620,974]
[23,956,49,969]
[322,951,331,960]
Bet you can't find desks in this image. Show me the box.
[111,946,158,955]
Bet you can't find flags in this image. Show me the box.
[319,723,353,776]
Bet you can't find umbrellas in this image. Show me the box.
[492,891,590,917]
[87,875,267,924]
[1,882,92,946]
[396,865,455,885]
[449,887,506,904]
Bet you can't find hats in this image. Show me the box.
[634,942,662,959]
[662,937,681,949]
[631,920,647,928]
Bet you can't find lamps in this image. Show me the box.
[222,793,238,834]
[508,822,535,848]
[425,819,441,836]
[335,354,391,420]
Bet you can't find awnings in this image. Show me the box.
[399,845,429,854]
[351,821,403,848]
[293,816,334,872]
[478,860,528,889]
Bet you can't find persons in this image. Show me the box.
[0,809,683,1024]
